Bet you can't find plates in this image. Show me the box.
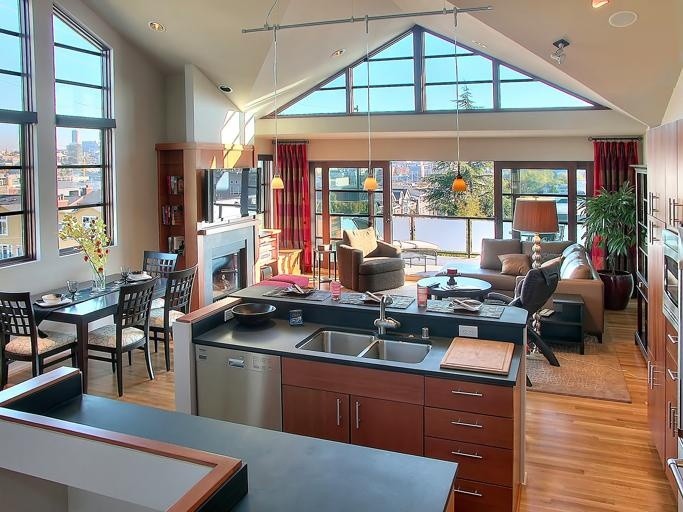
[359,293,391,305]
[283,285,312,296]
[446,299,483,312]
[34,296,72,307]
[127,274,153,281]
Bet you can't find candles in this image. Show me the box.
[447,268,457,274]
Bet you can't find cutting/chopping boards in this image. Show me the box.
[440,337,515,376]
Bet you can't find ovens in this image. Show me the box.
[665,434,683,512]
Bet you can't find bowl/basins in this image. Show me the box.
[231,303,277,323]
[41,294,63,304]
[130,270,146,278]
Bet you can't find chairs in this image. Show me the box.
[483,260,561,387]
[336,218,441,293]
[0,251,198,397]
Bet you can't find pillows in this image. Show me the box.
[480,239,592,280]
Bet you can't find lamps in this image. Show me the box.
[551,39,569,65]
[512,196,559,354]
[242,1,493,191]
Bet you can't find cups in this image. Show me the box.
[416,285,428,308]
[329,281,343,301]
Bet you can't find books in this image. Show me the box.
[162,175,185,257]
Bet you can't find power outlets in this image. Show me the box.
[459,325,478,338]
[225,309,234,321]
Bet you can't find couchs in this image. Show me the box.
[435,238,605,343]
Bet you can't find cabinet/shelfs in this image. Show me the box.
[539,293,584,355]
[629,120,683,503]
[155,142,303,312]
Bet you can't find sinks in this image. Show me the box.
[359,337,433,366]
[295,326,376,359]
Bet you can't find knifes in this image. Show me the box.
[452,299,478,311]
[293,284,305,294]
[365,291,382,302]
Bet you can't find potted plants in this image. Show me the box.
[571,181,636,311]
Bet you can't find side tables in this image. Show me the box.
[417,276,492,302]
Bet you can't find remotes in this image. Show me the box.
[427,282,440,288]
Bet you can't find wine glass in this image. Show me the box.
[66,281,78,303]
[121,265,130,286]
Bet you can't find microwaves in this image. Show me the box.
[661,244,681,326]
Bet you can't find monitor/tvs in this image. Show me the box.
[205,168,261,223]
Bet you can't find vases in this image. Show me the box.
[90,261,106,291]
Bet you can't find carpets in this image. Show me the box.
[526,326,632,403]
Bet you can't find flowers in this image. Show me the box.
[57,214,111,288]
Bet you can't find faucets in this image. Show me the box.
[366,289,401,334]
